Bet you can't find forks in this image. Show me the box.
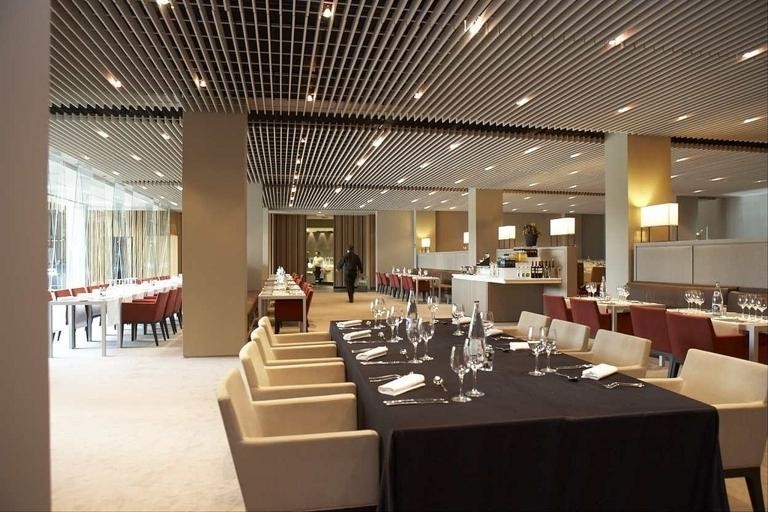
[601,382,644,389]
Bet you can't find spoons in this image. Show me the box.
[556,372,577,381]
[432,374,449,393]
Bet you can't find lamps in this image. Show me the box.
[639,203,679,243]
[420,238,431,253]
[462,231,470,252]
[497,225,517,250]
[549,217,576,246]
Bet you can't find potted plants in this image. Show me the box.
[519,221,542,247]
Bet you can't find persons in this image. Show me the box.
[306,251,310,262]
[313,251,324,283]
[336,246,364,303]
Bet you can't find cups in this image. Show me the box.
[481,344,494,371]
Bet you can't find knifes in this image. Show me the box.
[382,396,450,406]
[556,364,591,370]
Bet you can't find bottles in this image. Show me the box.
[467,298,491,365]
[498,247,560,279]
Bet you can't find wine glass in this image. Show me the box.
[528,327,547,376]
[542,329,558,374]
[465,337,489,399]
[361,288,440,366]
[452,345,472,402]
[451,303,465,337]
[585,275,630,302]
[481,311,494,329]
[683,280,768,321]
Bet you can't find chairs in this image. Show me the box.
[45,272,184,359]
